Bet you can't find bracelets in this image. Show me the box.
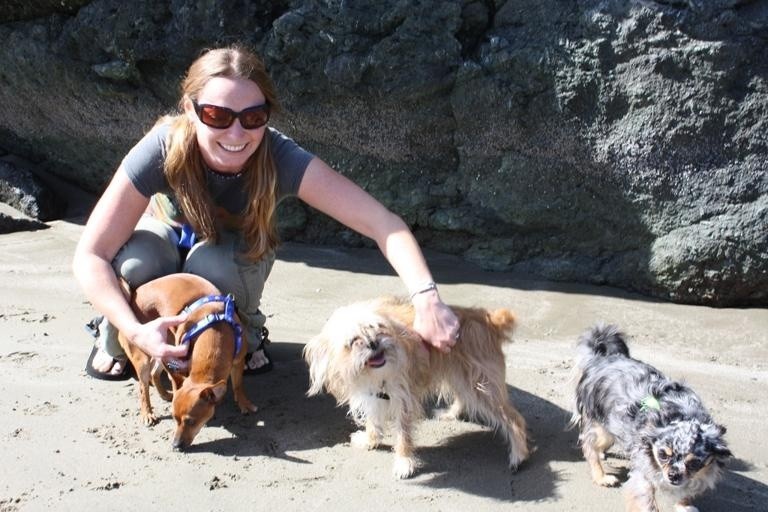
[408,280,438,299]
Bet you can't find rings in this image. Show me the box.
[443,346,452,353]
[455,332,460,340]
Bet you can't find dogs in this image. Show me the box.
[563,320,734,512]
[117,272,260,453]
[300,294,531,482]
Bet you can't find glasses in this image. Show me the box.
[191,99,269,129]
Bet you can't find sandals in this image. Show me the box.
[87,345,132,380]
[243,344,273,375]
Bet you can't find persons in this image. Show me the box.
[70,47,460,380]
